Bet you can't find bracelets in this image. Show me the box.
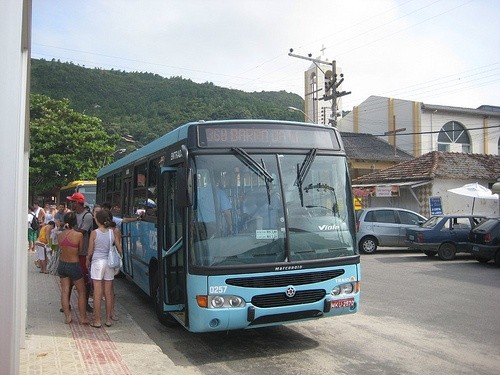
[136,217,140,221]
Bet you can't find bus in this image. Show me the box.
[57,180,97,213]
[94,118,363,335]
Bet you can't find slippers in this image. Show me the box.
[111,313,119,321]
[79,320,90,325]
[64,317,73,324]
[105,322,111,326]
[40,270,53,274]
[90,321,101,328]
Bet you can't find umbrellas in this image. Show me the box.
[447,181,499,215]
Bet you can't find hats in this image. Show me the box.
[49,205,56,210]
[67,192,85,204]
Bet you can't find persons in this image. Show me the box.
[110,204,159,217]
[58,213,89,325]
[89,203,143,321]
[85,210,122,328]
[194,167,232,242]
[60,192,93,313]
[252,191,279,230]
[28,201,71,274]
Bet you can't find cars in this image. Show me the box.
[467,215,500,269]
[405,213,489,262]
[356,206,442,254]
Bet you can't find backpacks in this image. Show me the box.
[29,213,39,230]
[82,211,98,230]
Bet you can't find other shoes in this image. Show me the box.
[88,298,94,303]
[101,296,106,302]
[86,304,94,313]
[60,305,71,312]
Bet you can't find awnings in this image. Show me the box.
[352,179,430,189]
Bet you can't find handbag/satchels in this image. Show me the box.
[107,229,120,267]
[77,229,90,256]
